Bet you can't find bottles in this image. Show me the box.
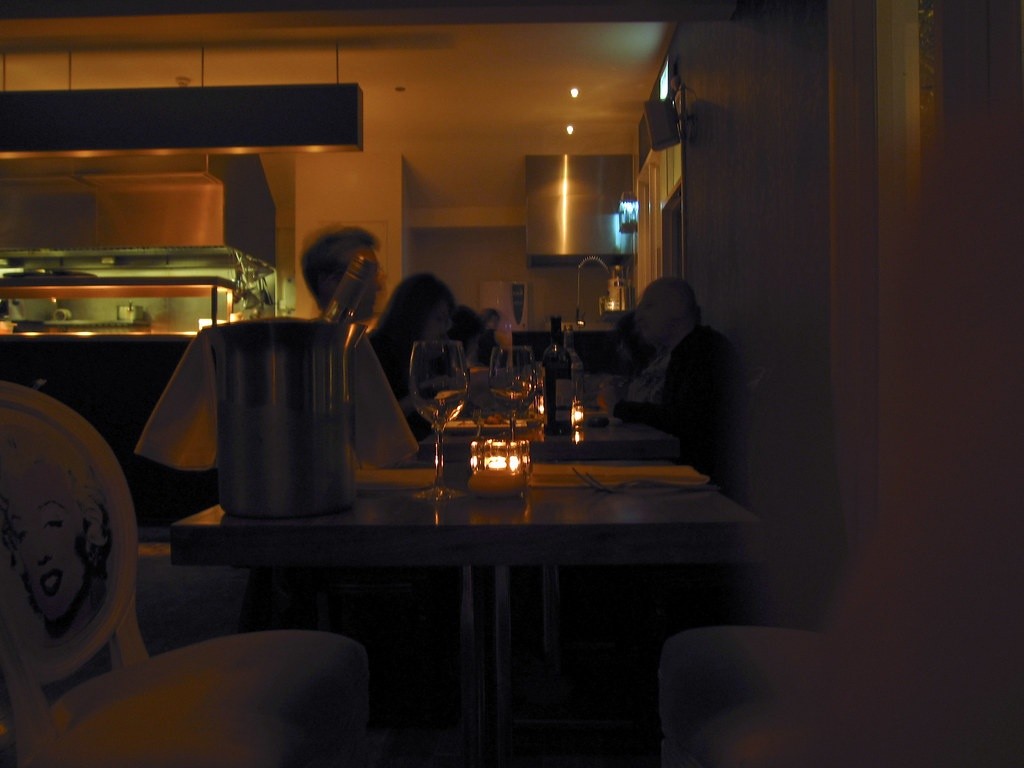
[562,324,583,424]
[542,315,573,435]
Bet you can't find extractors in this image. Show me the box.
[1,153,278,274]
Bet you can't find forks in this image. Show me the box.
[571,467,692,494]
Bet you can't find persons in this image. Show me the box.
[599,276,736,674]
[478,309,509,368]
[447,305,487,354]
[371,271,463,399]
[245,226,431,677]
[615,313,668,391]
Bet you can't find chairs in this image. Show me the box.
[1,378,369,768]
[655,87,1023,768]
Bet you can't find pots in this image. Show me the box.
[8,297,56,322]
[117,300,143,321]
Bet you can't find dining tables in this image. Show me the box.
[407,386,681,677]
[165,458,766,768]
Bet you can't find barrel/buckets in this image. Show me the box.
[202,319,369,519]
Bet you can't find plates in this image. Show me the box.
[354,468,437,488]
[429,419,526,431]
[529,464,710,486]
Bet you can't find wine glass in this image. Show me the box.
[408,338,471,500]
[468,341,545,440]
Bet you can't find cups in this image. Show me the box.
[471,439,529,498]
